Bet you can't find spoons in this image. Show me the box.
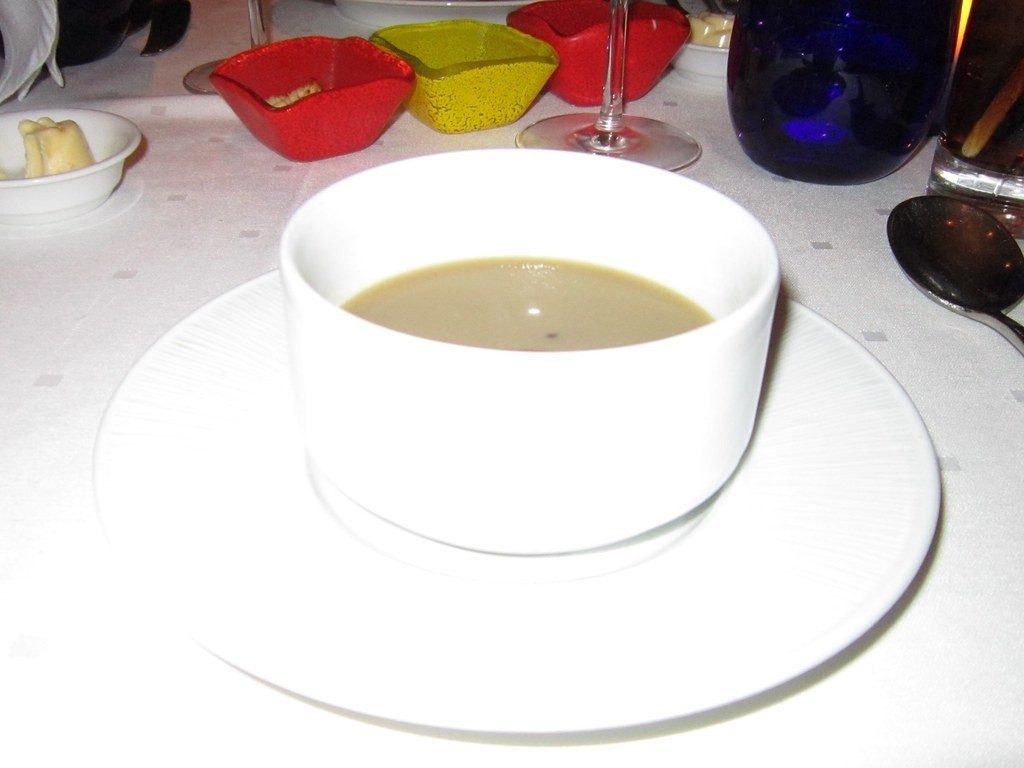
[886,194,1024,357]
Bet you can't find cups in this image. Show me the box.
[925,0,1024,239]
[728,0,963,185]
[280,147,782,557]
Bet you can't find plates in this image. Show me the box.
[361,0,539,12]
[0,170,144,241]
[89,264,941,737]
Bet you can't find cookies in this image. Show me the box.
[265,79,322,109]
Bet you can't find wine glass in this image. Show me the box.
[182,0,260,95]
[514,0,703,171]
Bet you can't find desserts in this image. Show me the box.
[17,118,96,180]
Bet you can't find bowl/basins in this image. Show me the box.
[208,35,417,163]
[505,0,693,107]
[0,107,142,225]
[369,18,560,135]
[671,43,729,87]
